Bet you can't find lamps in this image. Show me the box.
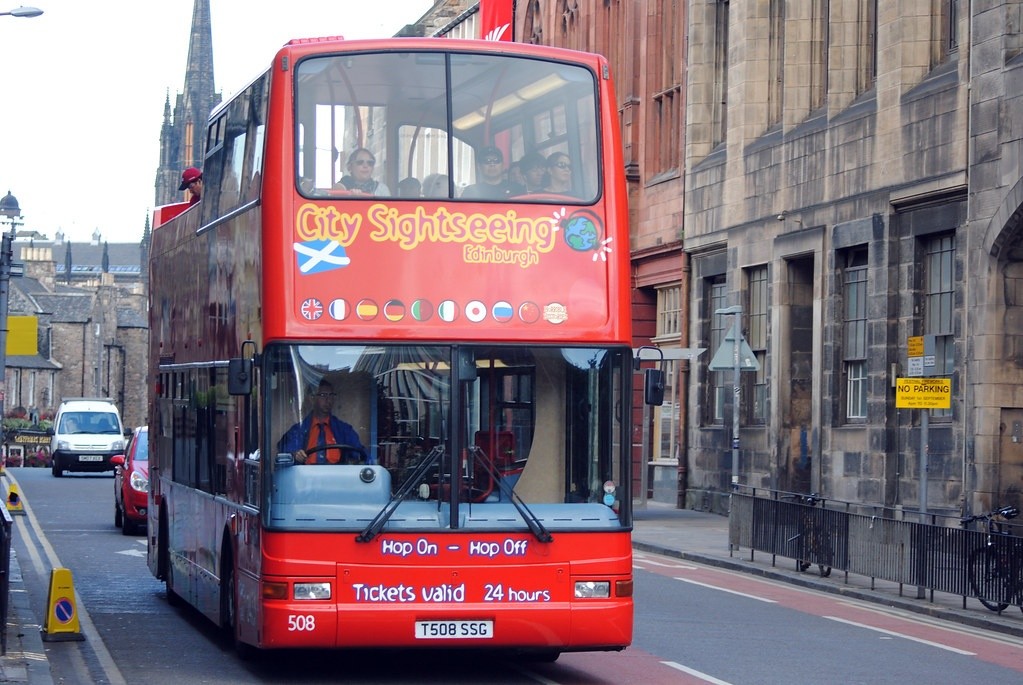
[777,211,802,224]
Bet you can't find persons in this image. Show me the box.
[459,146,573,199]
[178,168,202,205]
[397,173,456,196]
[278,380,375,465]
[333,145,391,196]
[66,417,81,433]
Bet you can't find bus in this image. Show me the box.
[146,36,668,668]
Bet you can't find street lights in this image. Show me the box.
[713,305,742,484]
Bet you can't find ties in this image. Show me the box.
[315,422,328,464]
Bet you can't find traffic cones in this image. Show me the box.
[4,482,29,516]
[40,568,86,643]
[1,461,10,475]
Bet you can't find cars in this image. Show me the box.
[109,424,150,535]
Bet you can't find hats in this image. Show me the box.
[179,168,202,190]
[478,147,504,163]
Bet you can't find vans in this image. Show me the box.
[47,397,131,478]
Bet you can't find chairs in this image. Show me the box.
[472,431,517,483]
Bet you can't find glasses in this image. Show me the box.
[311,393,338,400]
[352,159,374,168]
[479,157,501,164]
[551,161,573,172]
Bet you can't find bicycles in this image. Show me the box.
[961,505,1023,612]
[781,492,833,577]
